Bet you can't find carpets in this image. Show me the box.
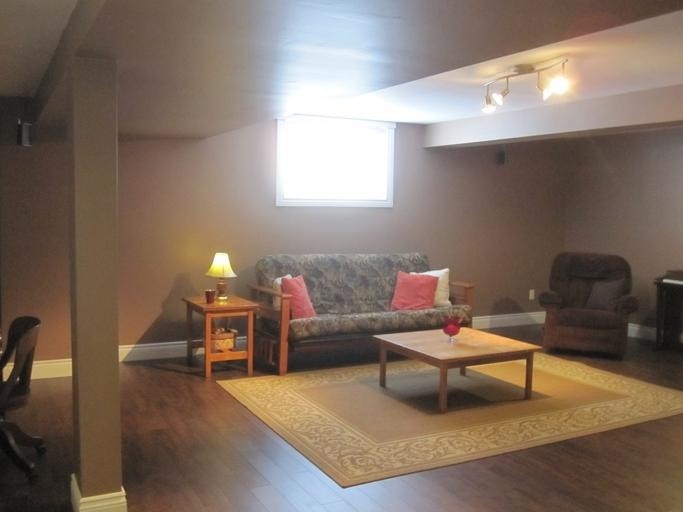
[216,352,683,488]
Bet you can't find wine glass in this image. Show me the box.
[442,315,462,344]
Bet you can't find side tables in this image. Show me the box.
[182,292,259,381]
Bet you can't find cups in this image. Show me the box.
[203,289,215,304]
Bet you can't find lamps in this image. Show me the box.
[480,58,569,114]
[205,252,239,300]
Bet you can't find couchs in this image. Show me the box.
[246,251,475,375]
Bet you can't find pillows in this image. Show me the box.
[281,273,317,320]
[583,278,627,312]
[272,274,293,311]
[409,268,454,308]
[390,271,438,311]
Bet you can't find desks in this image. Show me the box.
[653,278,683,354]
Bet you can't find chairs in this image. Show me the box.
[0,316,46,483]
[538,252,640,361]
